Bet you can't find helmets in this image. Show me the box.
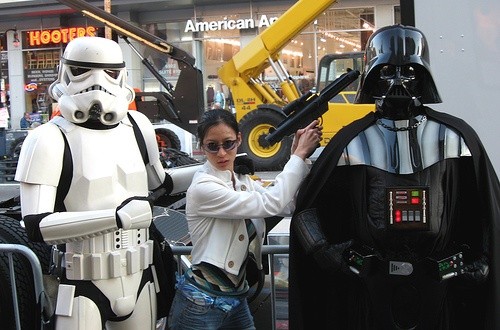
[48,37,135,125]
[351,24,444,106]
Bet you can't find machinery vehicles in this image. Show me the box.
[0,1,375,330]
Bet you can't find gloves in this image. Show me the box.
[233,157,255,176]
[118,196,154,230]
[294,207,362,274]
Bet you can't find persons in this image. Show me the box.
[289,24,500,330]
[20,112,30,128]
[167,108,323,330]
[14,36,255,330]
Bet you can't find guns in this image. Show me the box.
[264,67,362,159]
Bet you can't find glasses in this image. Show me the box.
[202,137,240,152]
